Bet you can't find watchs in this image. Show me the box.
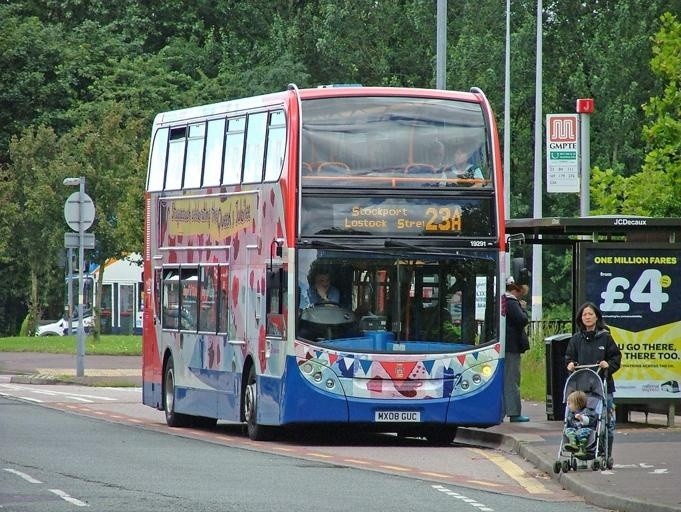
[321,295,328,300]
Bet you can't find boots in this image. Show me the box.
[574,438,588,457]
[564,432,579,452]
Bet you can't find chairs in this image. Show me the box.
[302,161,438,178]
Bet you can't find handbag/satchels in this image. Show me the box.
[520,328,530,354]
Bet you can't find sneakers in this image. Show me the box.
[510,415,529,422]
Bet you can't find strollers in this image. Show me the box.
[553,364,614,474]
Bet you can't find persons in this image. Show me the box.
[298,268,340,309]
[504,282,529,422]
[438,143,485,188]
[563,391,596,457]
[564,301,621,470]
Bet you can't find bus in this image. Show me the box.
[143,84,528,447]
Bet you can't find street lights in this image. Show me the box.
[63,176,85,377]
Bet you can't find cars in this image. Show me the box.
[34,316,91,336]
